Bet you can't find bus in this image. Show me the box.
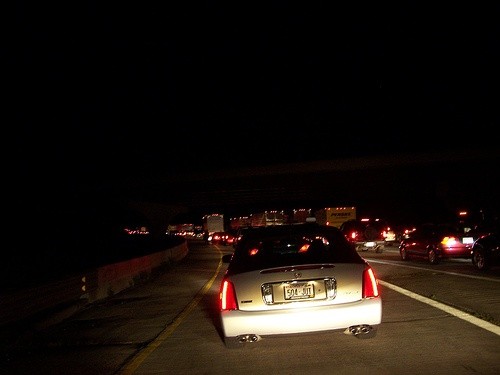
[204,206,357,235]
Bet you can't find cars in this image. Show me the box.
[382,225,395,244]
[340,219,387,255]
[122,223,243,246]
[470,232,500,274]
[400,229,474,262]
[220,224,381,345]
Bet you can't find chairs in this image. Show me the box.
[252,244,281,269]
[305,239,327,264]
